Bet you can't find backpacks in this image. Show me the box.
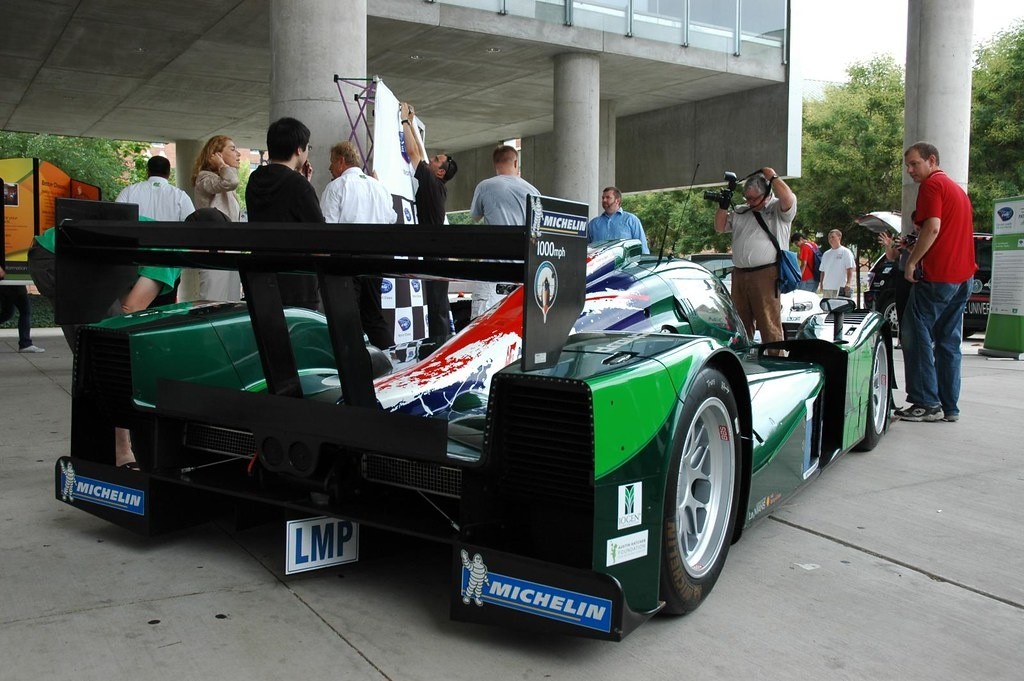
[804,242,823,282]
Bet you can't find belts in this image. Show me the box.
[735,262,777,273]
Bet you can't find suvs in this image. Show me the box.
[846,209,995,346]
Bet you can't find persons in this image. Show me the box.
[586,187,651,257]
[244,117,324,311]
[470,145,542,321]
[114,155,198,304]
[793,142,977,422]
[193,135,241,307]
[0,260,45,353]
[713,167,797,356]
[400,102,457,340]
[320,140,398,351]
[25,207,232,461]
[819,226,856,301]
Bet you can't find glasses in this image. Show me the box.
[447,155,452,173]
[306,143,312,151]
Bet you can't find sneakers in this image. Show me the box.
[894,404,945,421]
[18,345,45,353]
[944,413,959,422]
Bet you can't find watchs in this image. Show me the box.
[401,119,412,125]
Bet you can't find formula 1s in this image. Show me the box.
[53,193,899,643]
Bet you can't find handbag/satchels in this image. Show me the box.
[776,249,802,294]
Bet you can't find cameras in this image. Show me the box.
[904,232,918,245]
[704,171,737,209]
